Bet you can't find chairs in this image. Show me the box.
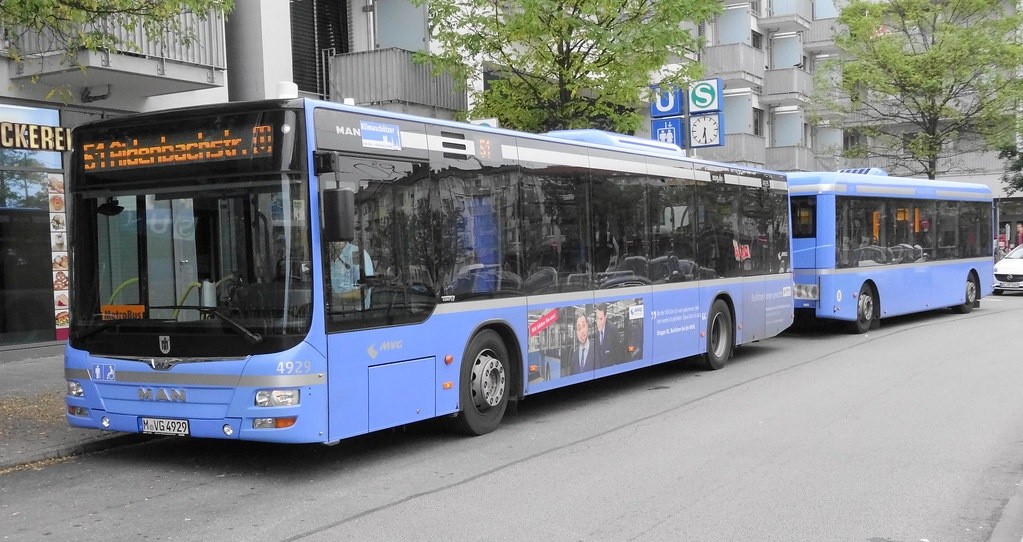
[526,267,558,286]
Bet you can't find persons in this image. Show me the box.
[570,313,601,375]
[329,241,374,310]
[1014,222,1023,247]
[595,302,620,368]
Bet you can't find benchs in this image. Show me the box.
[568,270,636,286]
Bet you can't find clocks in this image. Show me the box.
[688,110,725,149]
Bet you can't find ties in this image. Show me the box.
[601,330,603,343]
[581,347,585,368]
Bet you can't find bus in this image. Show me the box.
[785,168,994,334]
[65,98,796,445]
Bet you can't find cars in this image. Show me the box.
[994,244,1023,296]
